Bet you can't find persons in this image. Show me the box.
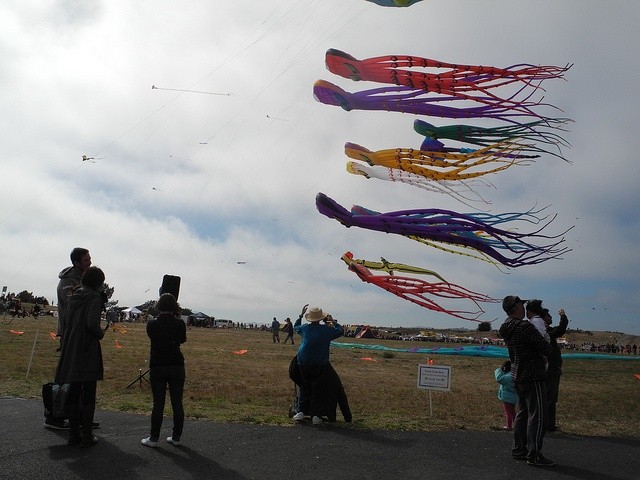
[141,295,186,448]
[526,299,546,337]
[543,309,568,430]
[61,266,108,446]
[500,295,556,466]
[440,332,499,346]
[44,247,97,429]
[294,303,344,425]
[271,317,280,343]
[495,361,516,431]
[283,318,294,344]
[568,342,640,355]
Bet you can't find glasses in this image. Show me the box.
[546,314,550,317]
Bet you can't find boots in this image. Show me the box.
[79,425,98,448]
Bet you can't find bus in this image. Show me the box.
[213,318,233,329]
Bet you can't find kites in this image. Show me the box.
[340,251,459,289]
[325,48,573,103]
[420,137,541,160]
[344,141,523,178]
[366,0,421,7]
[348,261,499,323]
[314,80,543,120]
[315,191,574,269]
[151,85,230,96]
[237,260,248,265]
[346,161,502,212]
[82,153,102,164]
[412,119,573,151]
[351,205,517,274]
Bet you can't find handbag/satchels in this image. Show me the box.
[43,383,75,419]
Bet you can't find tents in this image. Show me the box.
[121,306,141,321]
[356,327,379,338]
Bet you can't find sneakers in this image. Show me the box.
[141,437,158,447]
[511,451,529,459]
[312,416,322,424]
[293,412,304,421]
[527,453,557,466]
[44,417,71,430]
[166,437,181,446]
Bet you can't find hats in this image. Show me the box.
[305,307,327,322]
[503,296,530,313]
